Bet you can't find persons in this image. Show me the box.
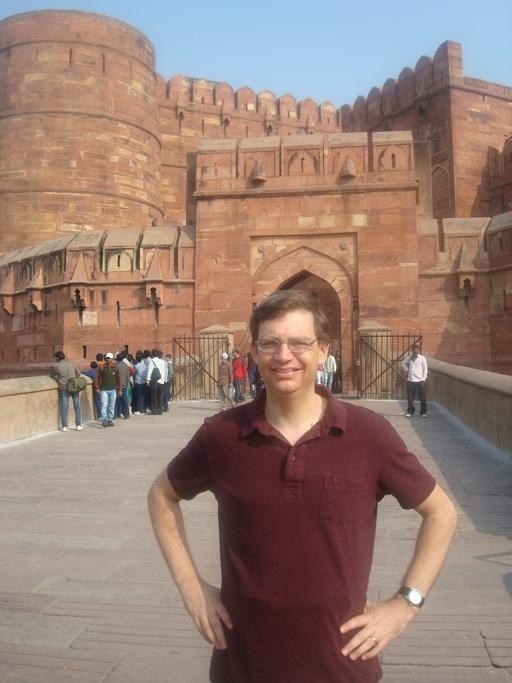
[148,288,459,683]
[317,347,342,393]
[400,344,429,417]
[51,349,174,433]
[216,350,264,412]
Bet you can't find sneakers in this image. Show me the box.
[76,423,84,430]
[422,414,427,418]
[405,413,413,418]
[62,425,68,432]
[102,419,114,426]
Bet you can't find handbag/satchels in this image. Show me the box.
[67,376,88,394]
[129,366,136,376]
[150,368,161,385]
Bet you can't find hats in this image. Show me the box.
[106,352,114,358]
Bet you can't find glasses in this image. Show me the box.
[254,335,319,353]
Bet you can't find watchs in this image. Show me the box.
[396,585,427,609]
